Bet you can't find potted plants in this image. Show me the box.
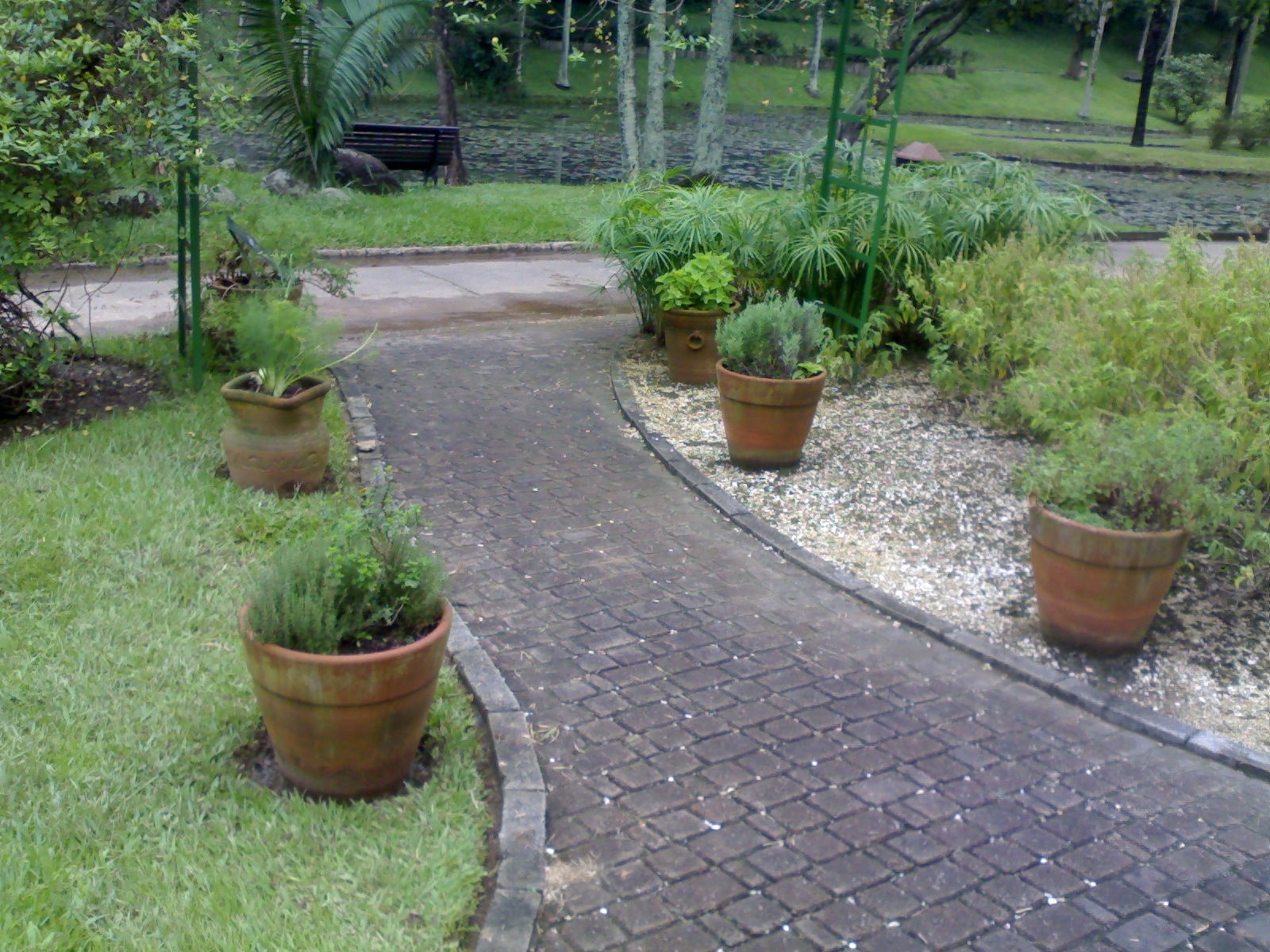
[148,163,360,348]
[198,287,383,495]
[655,250,741,384]
[712,287,833,471]
[997,292,1268,657]
[238,464,454,798]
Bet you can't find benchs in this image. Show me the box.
[341,123,460,188]
